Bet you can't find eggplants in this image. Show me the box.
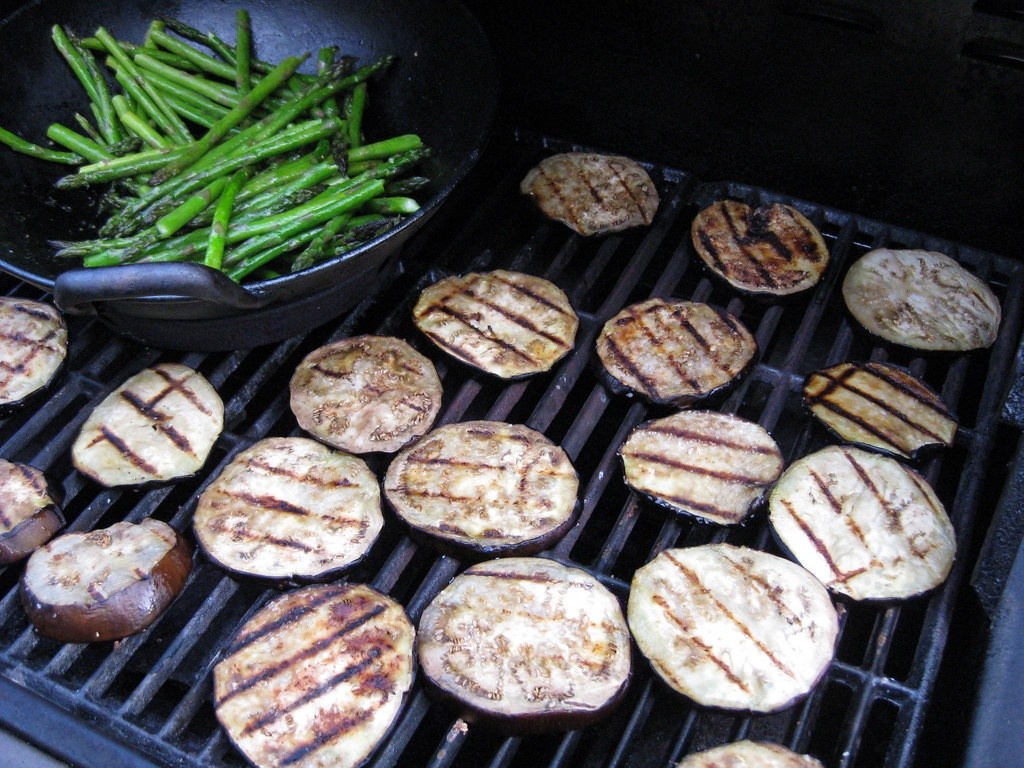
[0,151,1003,768]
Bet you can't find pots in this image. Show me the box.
[0,1,498,319]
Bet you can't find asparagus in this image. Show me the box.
[0,11,436,284]
